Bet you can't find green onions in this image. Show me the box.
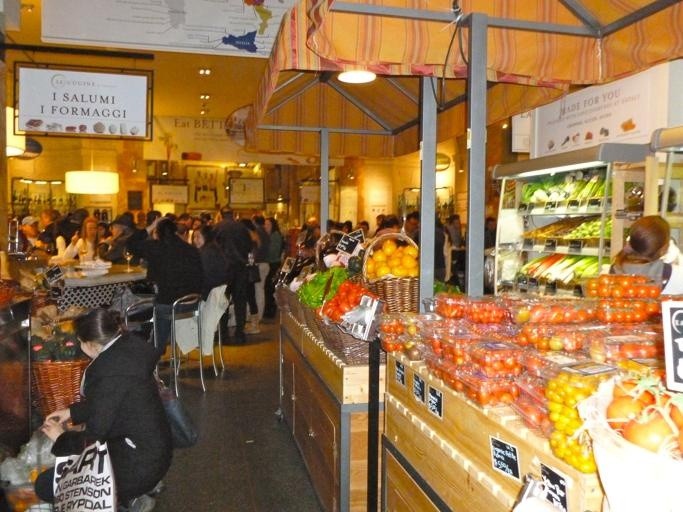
[537,255,598,290]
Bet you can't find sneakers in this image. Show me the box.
[117,480,166,512]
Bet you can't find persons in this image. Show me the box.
[32,308,175,511]
[327,211,496,283]
[17,205,162,291]
[125,217,210,357]
[164,204,320,337]
[608,213,683,296]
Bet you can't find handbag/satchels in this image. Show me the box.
[53,440,116,511]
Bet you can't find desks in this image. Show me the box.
[378,351,605,512]
[278,308,386,512]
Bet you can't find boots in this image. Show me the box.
[244,313,264,335]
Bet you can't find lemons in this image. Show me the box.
[366,239,419,278]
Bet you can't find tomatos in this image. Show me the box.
[379,275,683,474]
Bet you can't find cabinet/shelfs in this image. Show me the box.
[490,142,682,298]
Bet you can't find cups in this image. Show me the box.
[353,295,386,342]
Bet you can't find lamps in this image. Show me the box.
[63,138,119,196]
[5,106,26,157]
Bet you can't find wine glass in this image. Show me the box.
[122,249,135,272]
